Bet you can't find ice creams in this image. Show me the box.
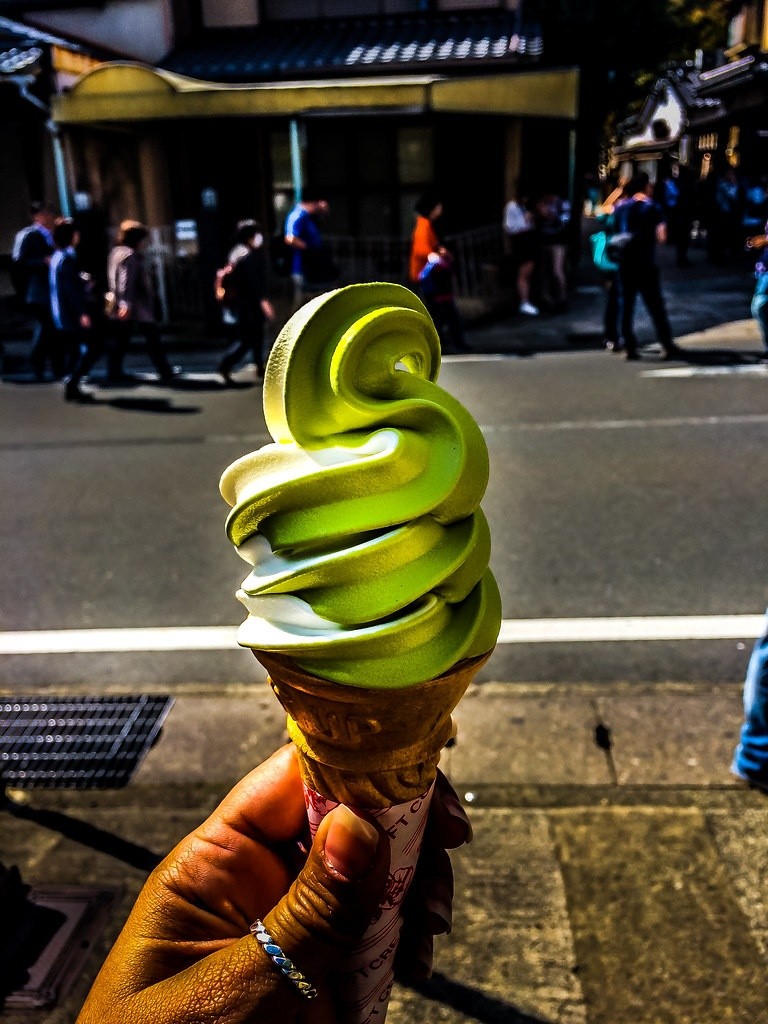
[218,278,503,812]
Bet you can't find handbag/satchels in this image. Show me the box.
[104,291,132,321]
[591,230,620,272]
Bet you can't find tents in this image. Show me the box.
[50,64,578,224]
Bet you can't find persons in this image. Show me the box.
[728,632,768,786]
[11,164,767,404]
[74,744,473,1024]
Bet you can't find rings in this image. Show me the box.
[251,919,316,998]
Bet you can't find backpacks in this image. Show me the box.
[214,250,251,301]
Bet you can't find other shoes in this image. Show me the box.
[163,366,182,386]
[521,302,540,315]
[218,362,235,384]
[63,392,96,406]
